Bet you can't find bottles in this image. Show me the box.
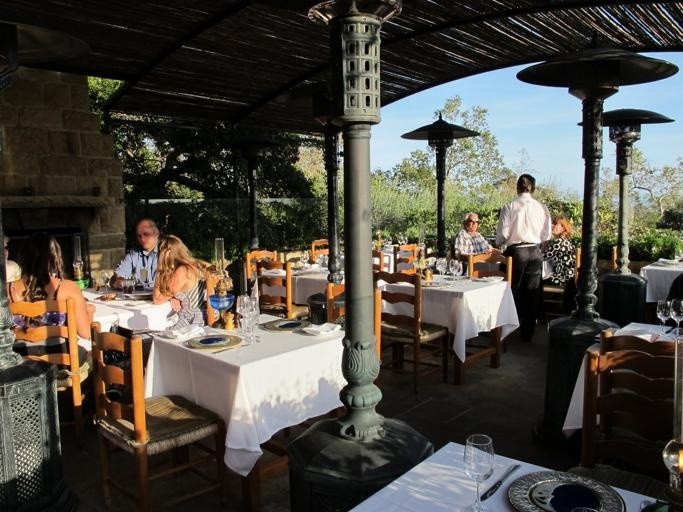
[423,266,433,285]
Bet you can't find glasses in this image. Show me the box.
[466,220,480,224]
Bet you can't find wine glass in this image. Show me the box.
[299,252,344,277]
[662,439,683,493]
[236,294,260,346]
[101,271,111,293]
[463,433,496,512]
[670,299,683,342]
[436,257,463,286]
[656,301,671,341]
[384,231,409,246]
[208,293,235,328]
[140,266,149,293]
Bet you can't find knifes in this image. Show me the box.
[479,463,520,502]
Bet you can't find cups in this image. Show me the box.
[120,279,136,294]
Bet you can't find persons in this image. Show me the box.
[12,232,95,422]
[3,229,20,303]
[140,233,210,365]
[109,217,161,353]
[453,211,495,262]
[492,174,552,341]
[534,215,576,323]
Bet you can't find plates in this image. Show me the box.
[472,275,505,283]
[506,470,628,512]
[444,275,471,281]
[264,319,312,332]
[188,334,242,351]
